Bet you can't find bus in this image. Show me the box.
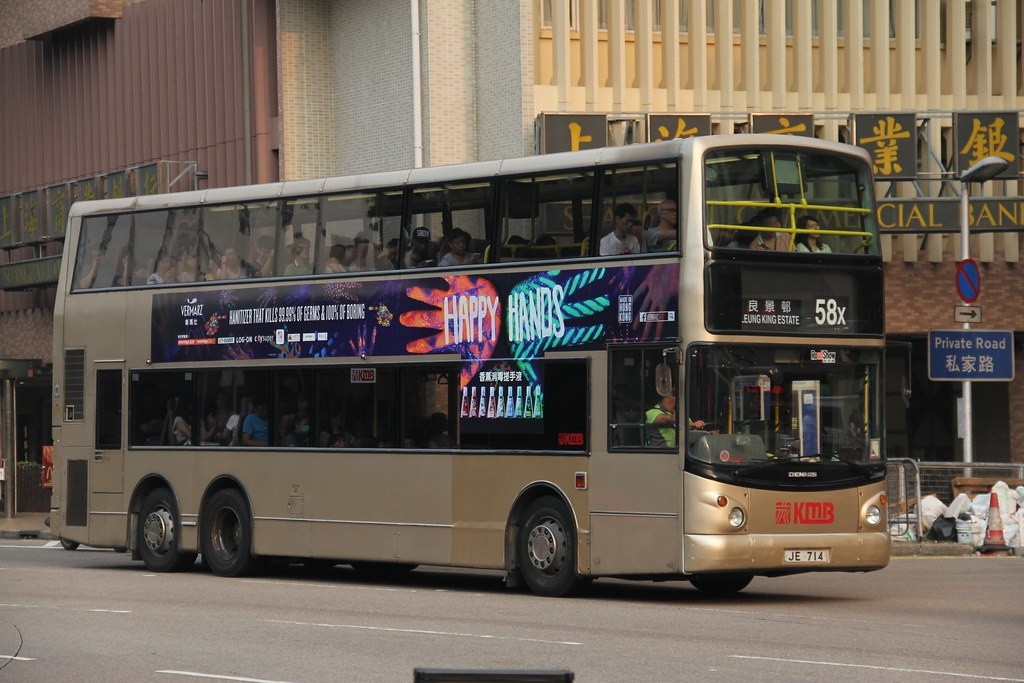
[50,133,913,596]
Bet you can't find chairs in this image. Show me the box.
[353,233,593,281]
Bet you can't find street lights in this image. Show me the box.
[961,156,1008,493]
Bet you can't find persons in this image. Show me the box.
[645,395,705,455]
[626,262,681,342]
[138,389,455,448]
[75,235,315,287]
[728,207,832,256]
[598,198,676,256]
[324,228,556,275]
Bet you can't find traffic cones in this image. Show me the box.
[981,492,1014,551]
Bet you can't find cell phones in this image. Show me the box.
[425,260,433,263]
[473,252,481,256]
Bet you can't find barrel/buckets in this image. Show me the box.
[956,521,981,545]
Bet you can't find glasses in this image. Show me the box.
[662,208,676,212]
